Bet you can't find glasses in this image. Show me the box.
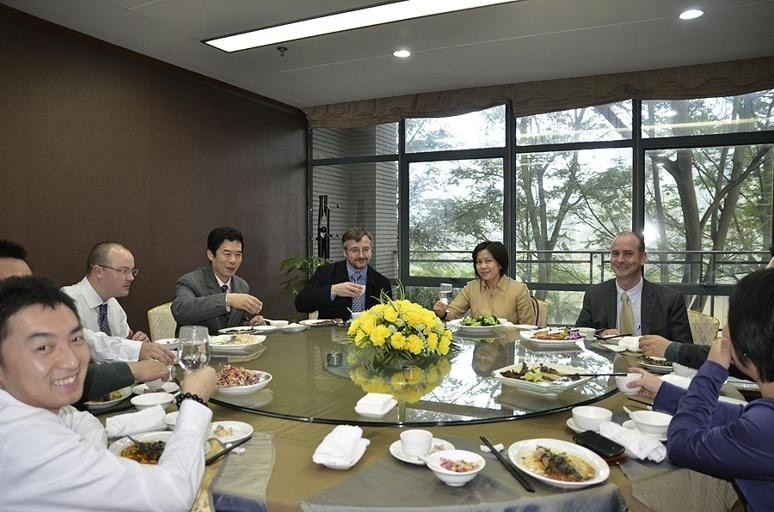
[346,247,372,254]
[97,264,139,277]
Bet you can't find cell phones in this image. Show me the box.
[572,429,626,459]
[603,453,627,466]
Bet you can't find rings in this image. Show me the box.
[646,350,652,356]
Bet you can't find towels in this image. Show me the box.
[598,423,669,465]
[104,405,168,438]
[312,425,365,467]
[617,335,643,352]
[660,372,691,390]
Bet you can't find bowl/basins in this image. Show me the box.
[629,408,673,435]
[130,392,174,411]
[571,405,612,428]
[614,371,643,398]
[164,411,180,429]
[601,335,621,344]
[351,312,362,318]
[426,450,485,487]
[270,320,289,327]
[154,338,180,350]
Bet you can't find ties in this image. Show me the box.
[621,292,633,336]
[351,272,362,315]
[97,304,112,337]
[221,285,228,324]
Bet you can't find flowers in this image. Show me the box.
[348,351,452,404]
[347,299,453,357]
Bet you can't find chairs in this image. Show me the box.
[529,297,549,328]
[309,310,319,320]
[683,310,719,346]
[146,300,177,341]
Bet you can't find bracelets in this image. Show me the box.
[176,392,208,409]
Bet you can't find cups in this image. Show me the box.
[359,285,365,296]
[576,326,596,338]
[145,378,163,389]
[400,428,433,459]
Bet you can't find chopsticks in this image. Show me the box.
[627,397,652,408]
[205,436,252,467]
[92,405,135,417]
[601,333,633,340]
[479,435,536,494]
[564,373,628,377]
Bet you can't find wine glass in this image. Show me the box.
[439,282,454,312]
[177,325,212,370]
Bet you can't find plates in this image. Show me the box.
[354,399,398,418]
[519,327,587,348]
[211,334,267,350]
[639,358,678,372]
[108,430,173,465]
[566,418,619,433]
[253,324,276,332]
[218,326,258,334]
[602,344,626,352]
[507,437,610,491]
[282,323,306,332]
[215,388,273,408]
[622,421,668,443]
[323,439,368,471]
[583,337,598,343]
[451,318,507,333]
[212,346,267,358]
[517,342,585,354]
[83,386,132,410]
[214,368,272,395]
[389,439,456,465]
[451,330,506,339]
[492,361,593,396]
[493,388,590,412]
[133,382,179,393]
[299,319,339,327]
[204,420,254,443]
[513,325,538,330]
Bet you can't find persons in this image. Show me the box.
[627,267,774,512]
[432,241,536,325]
[1,239,172,410]
[309,324,355,347]
[638,246,774,381]
[471,329,530,377]
[170,227,266,338]
[295,227,391,321]
[1,274,219,512]
[575,230,694,345]
[60,240,176,365]
